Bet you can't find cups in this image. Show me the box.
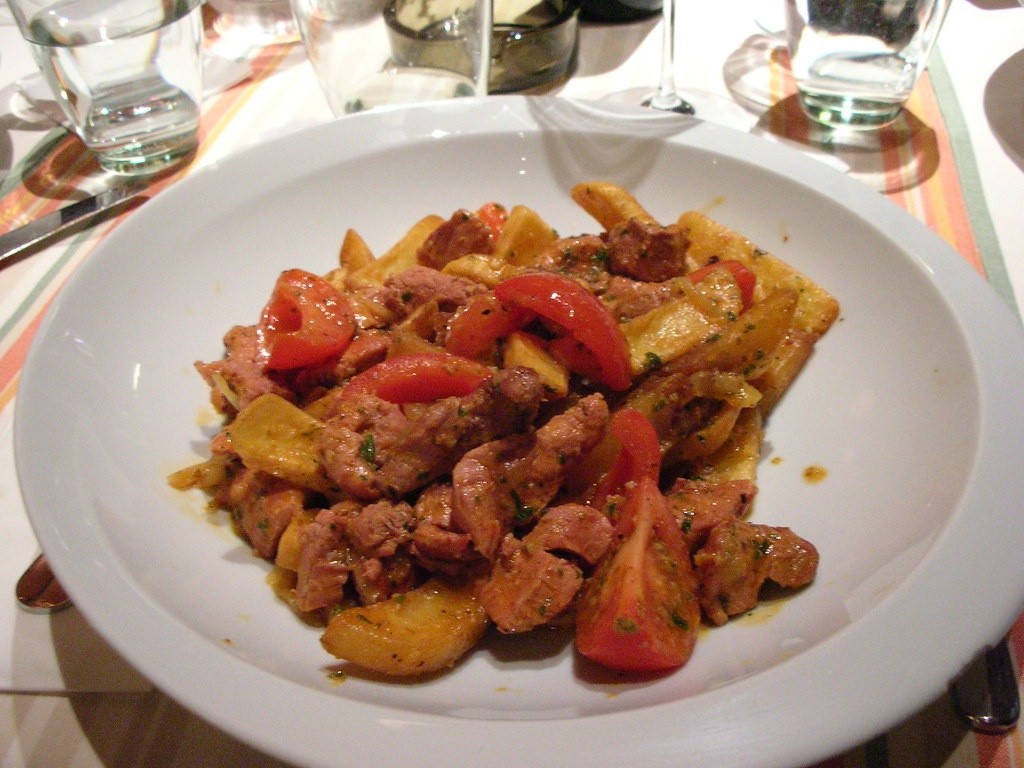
[207,0,301,45]
[291,0,490,117]
[7,0,204,175]
[785,0,952,131]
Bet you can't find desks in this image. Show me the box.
[0,0,1024,768]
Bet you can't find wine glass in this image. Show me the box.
[602,0,742,127]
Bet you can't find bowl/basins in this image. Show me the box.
[381,0,582,95]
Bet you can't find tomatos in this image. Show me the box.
[262,253,758,673]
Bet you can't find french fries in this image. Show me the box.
[228,183,838,678]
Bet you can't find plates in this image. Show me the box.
[12,98,1024,768]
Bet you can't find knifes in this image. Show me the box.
[0,182,148,261]
